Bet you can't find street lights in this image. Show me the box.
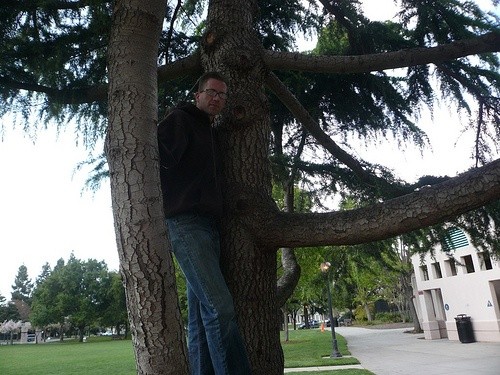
[320,262,342,359]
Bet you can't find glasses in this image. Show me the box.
[202,89,228,100]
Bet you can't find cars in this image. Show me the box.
[297,318,320,330]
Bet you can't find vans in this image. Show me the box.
[27,334,43,342]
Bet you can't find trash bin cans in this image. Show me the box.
[454,314,475,343]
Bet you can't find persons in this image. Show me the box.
[156,72,254,375]
